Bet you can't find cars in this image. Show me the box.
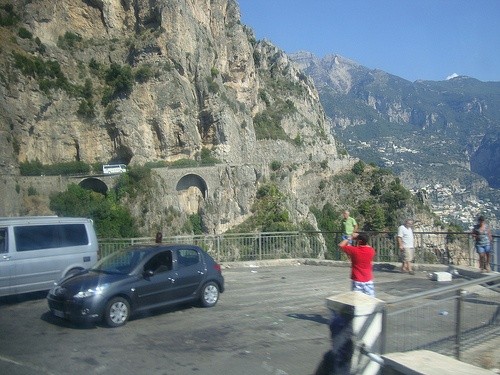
[47,242,226,329]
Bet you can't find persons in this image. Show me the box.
[397,219,416,275]
[338,210,358,262]
[472,216,492,275]
[338,232,375,296]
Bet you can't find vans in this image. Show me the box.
[0,216,99,298]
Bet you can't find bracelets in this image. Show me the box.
[347,238,351,240]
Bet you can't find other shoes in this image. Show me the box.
[409,271,417,275]
[401,268,409,272]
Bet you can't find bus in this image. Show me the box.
[102,164,126,174]
